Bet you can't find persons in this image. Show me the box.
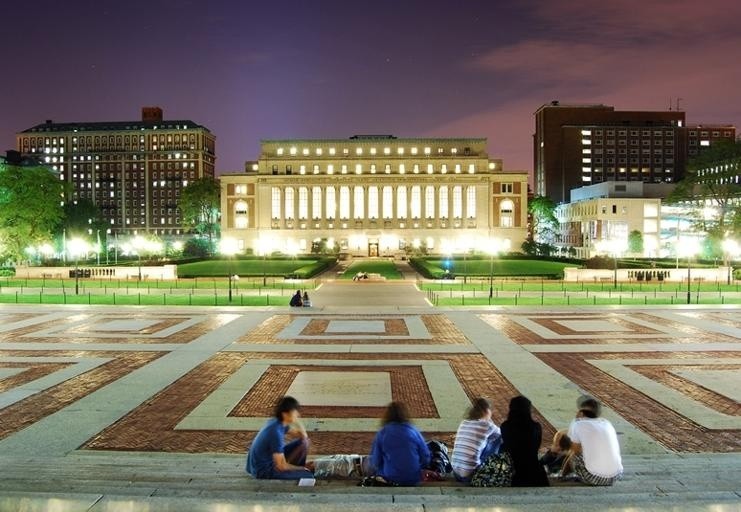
[301,291,312,307]
[551,392,624,485]
[449,396,503,483]
[290,290,302,307]
[246,395,316,480]
[358,400,431,487]
[539,426,574,478]
[498,395,552,486]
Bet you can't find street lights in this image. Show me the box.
[680,242,697,303]
[597,238,630,286]
[68,238,87,294]
[484,239,502,297]
[218,237,236,302]
[105,228,113,264]
[132,235,146,281]
[457,238,473,281]
[257,237,273,288]
[722,238,741,285]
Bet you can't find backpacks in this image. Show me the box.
[422,439,453,480]
[469,452,515,487]
